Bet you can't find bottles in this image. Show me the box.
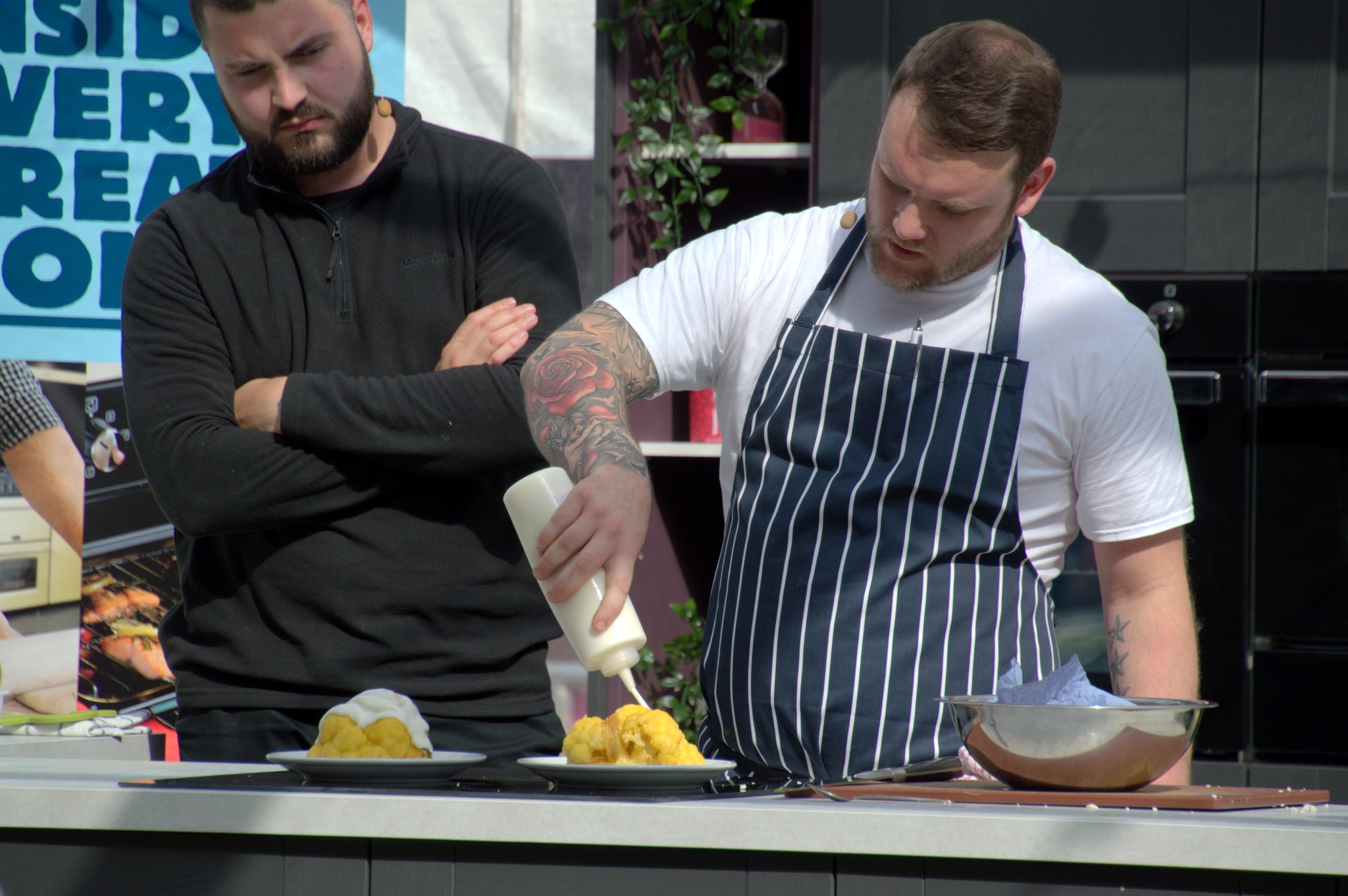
[503,466,648,694]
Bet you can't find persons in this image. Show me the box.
[522,18,1202,793]
[119,0,579,759]
[0,359,86,639]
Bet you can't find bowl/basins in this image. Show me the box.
[939,692,1220,790]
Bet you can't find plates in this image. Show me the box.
[265,752,486,782]
[515,755,737,787]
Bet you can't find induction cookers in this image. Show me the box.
[120,769,855,801]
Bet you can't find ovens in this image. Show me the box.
[1053,271,1348,768]
[0,539,48,613]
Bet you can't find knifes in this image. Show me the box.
[775,754,966,792]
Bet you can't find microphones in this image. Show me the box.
[371,72,392,117]
[840,187,867,229]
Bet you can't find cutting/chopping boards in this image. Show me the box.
[787,775,1332,811]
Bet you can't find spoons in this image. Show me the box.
[807,782,954,805]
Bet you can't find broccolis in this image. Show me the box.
[562,704,705,765]
[307,714,431,758]
